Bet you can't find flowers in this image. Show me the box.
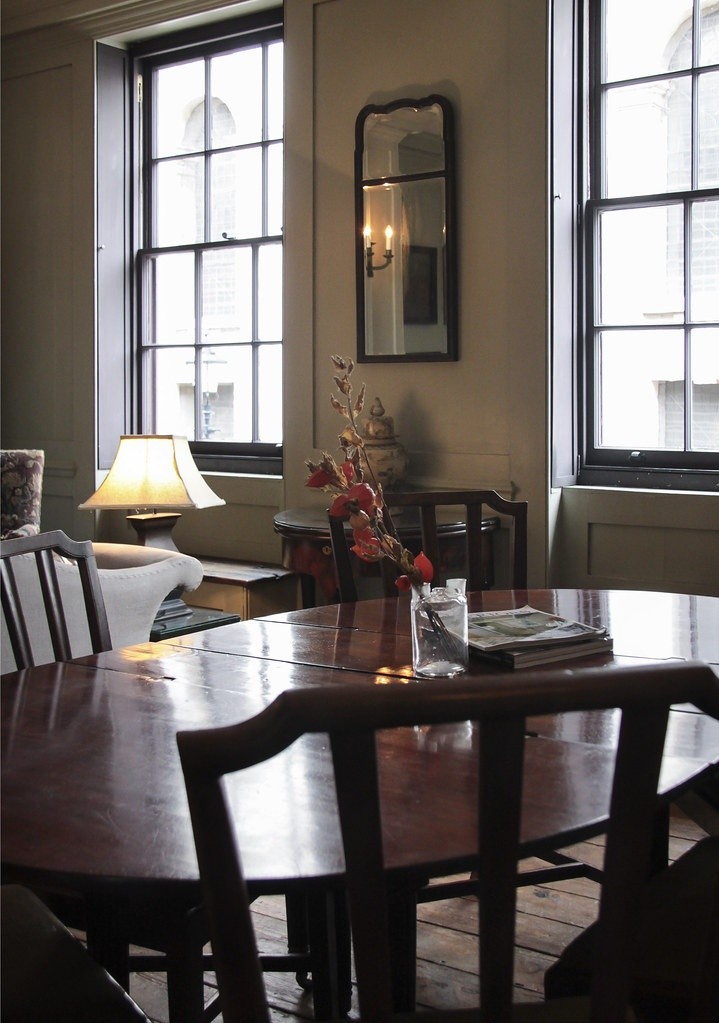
[304,353,436,588]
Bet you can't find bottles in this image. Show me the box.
[410,583,469,676]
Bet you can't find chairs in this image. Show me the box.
[0,529,314,1023]
[0,882,155,1023]
[174,658,719,1023]
[329,589,531,680]
[327,487,529,600]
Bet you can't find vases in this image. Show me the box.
[360,397,411,515]
[409,584,468,677]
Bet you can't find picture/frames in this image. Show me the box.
[402,245,439,325]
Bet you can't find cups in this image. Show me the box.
[445,579,466,595]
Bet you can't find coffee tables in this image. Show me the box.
[150,606,240,643]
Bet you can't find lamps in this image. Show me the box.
[78,433,225,553]
[363,223,394,278]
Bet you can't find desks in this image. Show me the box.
[180,556,297,621]
[0,588,713,1023]
[271,500,501,610]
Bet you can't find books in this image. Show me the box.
[420,606,614,669]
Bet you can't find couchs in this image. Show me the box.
[0,449,45,541]
[0,541,204,677]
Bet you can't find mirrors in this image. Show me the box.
[353,92,463,365]
